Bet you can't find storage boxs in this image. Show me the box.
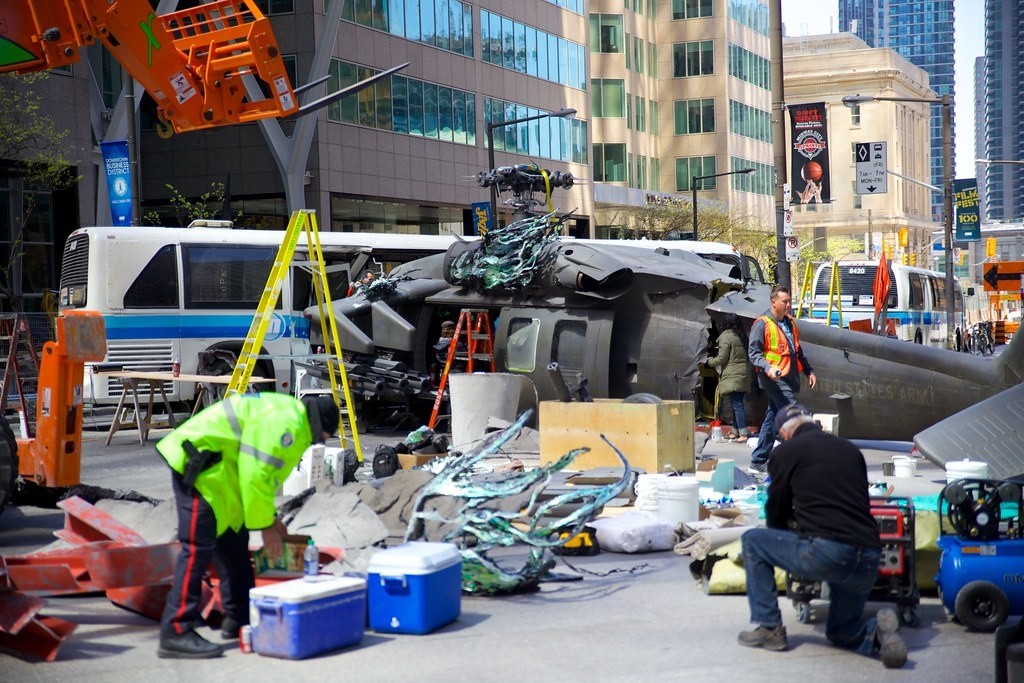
[249,574,368,661]
[368,540,462,634]
[539,400,695,473]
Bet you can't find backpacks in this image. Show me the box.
[372,444,403,479]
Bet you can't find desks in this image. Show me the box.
[93,364,278,447]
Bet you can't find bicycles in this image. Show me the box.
[966,320,996,357]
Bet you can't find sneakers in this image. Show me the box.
[750,462,767,473]
[876,608,907,668]
[738,626,789,651]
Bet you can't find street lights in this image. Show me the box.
[693,167,757,241]
[840,93,956,351]
[490,106,576,230]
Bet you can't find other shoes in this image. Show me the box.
[724,435,736,439]
[731,439,747,443]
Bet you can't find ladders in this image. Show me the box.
[428,309,496,430]
[0,313,39,438]
[224,210,364,466]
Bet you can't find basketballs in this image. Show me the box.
[801,162,822,182]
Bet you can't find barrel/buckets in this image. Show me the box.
[731,502,759,526]
[634,474,700,525]
[449,374,520,448]
[728,485,757,504]
[882,455,917,477]
[945,459,988,488]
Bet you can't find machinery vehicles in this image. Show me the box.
[0,0,409,504]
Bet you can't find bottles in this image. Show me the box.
[711,420,722,443]
[173,360,180,377]
[304,540,319,583]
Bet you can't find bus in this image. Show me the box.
[808,258,975,352]
[58,219,779,414]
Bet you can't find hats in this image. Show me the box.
[301,396,340,437]
[774,403,810,433]
[441,320,456,330]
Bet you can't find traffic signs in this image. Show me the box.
[855,141,888,195]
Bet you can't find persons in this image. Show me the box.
[154,393,340,660]
[748,286,817,475]
[433,321,465,432]
[347,270,375,297]
[739,404,908,670]
[795,179,822,203]
[707,315,751,443]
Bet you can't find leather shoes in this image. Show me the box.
[157,628,224,658]
[221,615,239,639]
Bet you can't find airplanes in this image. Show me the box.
[301,236,1024,482]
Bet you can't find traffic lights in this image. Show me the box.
[910,253,915,267]
[902,253,908,265]
[887,244,894,259]
